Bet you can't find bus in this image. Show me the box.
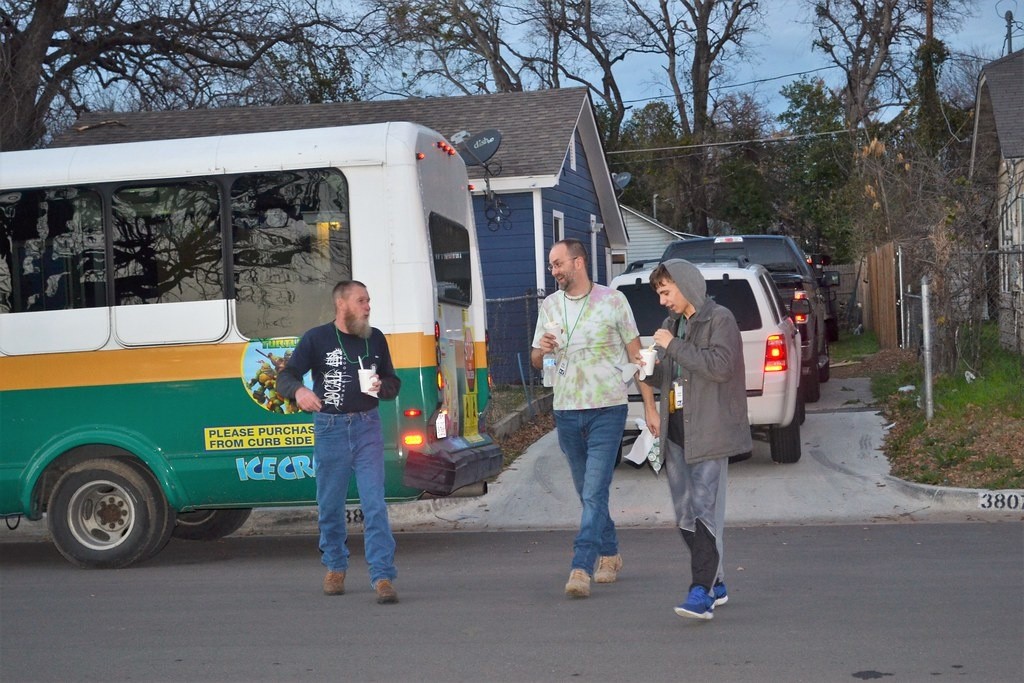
[1,118,502,570]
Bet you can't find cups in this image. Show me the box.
[544,322,562,346]
[639,349,657,376]
[358,369,375,393]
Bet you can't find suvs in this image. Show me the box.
[610,254,806,464]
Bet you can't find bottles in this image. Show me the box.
[543,353,556,387]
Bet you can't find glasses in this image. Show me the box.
[547,256,578,271]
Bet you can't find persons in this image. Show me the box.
[635,258,753,620]
[277,280,402,604]
[531,238,660,598]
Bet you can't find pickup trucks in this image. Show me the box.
[658,233,829,403]
[804,250,841,341]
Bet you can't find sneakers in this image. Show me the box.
[593,553,623,583]
[565,569,592,597]
[713,584,729,606]
[674,585,717,619]
[374,579,400,604]
[321,570,346,594]
